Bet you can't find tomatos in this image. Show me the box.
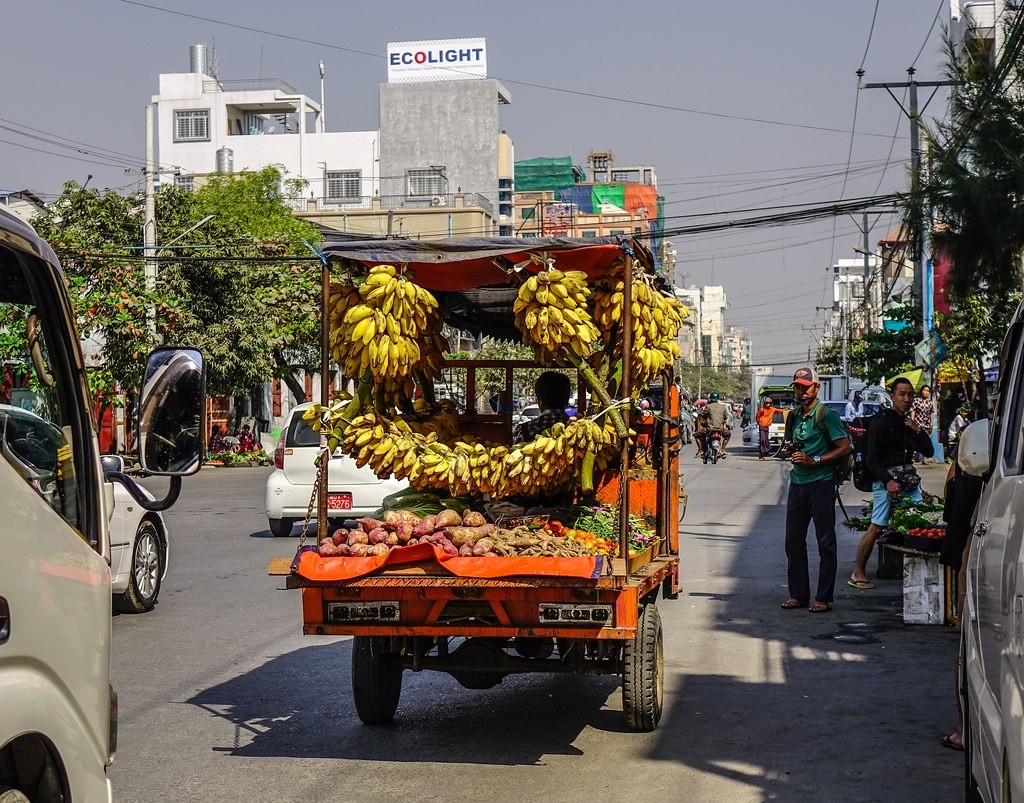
[534,520,637,557]
[911,528,945,538]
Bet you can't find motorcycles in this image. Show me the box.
[286,231,682,732]
[698,428,722,464]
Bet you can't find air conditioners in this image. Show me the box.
[432,196,444,206]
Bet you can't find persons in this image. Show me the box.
[845,392,864,449]
[207,425,235,453]
[971,477,995,590]
[909,385,935,465]
[147,410,200,472]
[938,419,992,749]
[948,407,971,442]
[693,392,731,458]
[736,397,751,442]
[779,367,850,612]
[25,426,51,471]
[639,389,658,410]
[236,424,256,452]
[675,377,685,421]
[755,398,795,460]
[513,371,578,445]
[847,378,934,590]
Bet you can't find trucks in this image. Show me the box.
[751,372,893,461]
[0,203,212,801]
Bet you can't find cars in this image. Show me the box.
[266,401,412,536]
[955,296,1022,802]
[0,403,171,615]
[742,398,888,451]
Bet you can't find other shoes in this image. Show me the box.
[721,452,726,459]
[695,450,703,457]
[922,462,929,465]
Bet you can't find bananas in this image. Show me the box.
[513,269,691,390]
[326,265,451,417]
[304,389,638,499]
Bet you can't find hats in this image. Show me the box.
[764,397,771,402]
[854,393,863,398]
[789,367,819,387]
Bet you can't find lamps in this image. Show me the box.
[892,285,914,303]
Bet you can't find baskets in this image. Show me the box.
[903,525,947,551]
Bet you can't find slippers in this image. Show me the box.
[809,606,832,613]
[781,600,810,609]
[941,733,964,751]
[848,580,875,589]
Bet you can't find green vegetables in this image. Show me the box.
[202,450,273,463]
[841,492,944,531]
[370,484,488,519]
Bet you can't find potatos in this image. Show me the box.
[317,509,497,557]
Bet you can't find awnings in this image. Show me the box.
[885,369,923,390]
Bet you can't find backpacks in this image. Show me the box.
[790,404,854,521]
[853,410,890,492]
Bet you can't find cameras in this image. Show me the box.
[784,442,805,465]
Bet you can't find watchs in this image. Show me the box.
[813,455,821,464]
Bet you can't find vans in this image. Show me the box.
[633,382,695,447]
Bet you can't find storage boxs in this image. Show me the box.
[879,532,956,626]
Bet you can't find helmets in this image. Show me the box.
[709,392,719,401]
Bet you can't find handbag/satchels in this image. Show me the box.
[886,464,921,492]
[938,441,983,531]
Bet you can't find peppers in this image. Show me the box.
[546,501,666,551]
